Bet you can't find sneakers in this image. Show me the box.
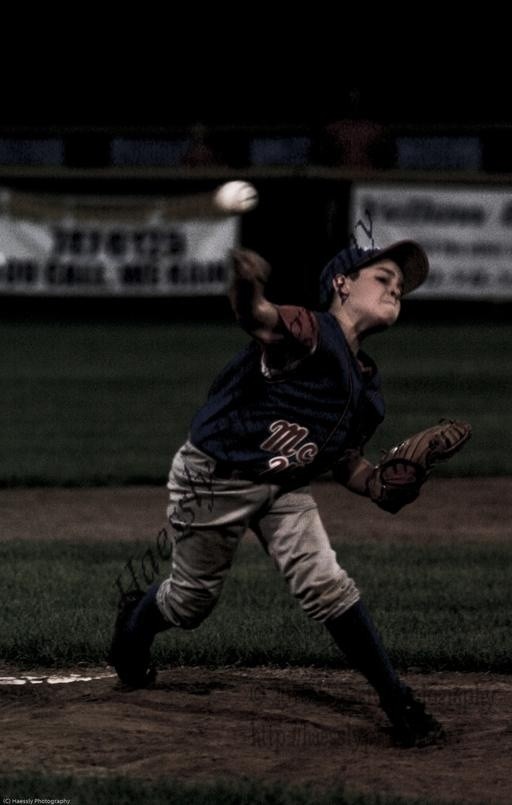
[381,681,446,749]
[109,591,157,686]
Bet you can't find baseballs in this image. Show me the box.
[216,181,257,212]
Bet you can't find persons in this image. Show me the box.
[110,238,471,738]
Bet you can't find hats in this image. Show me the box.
[320,238,429,309]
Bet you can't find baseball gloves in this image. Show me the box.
[366,419,473,514]
